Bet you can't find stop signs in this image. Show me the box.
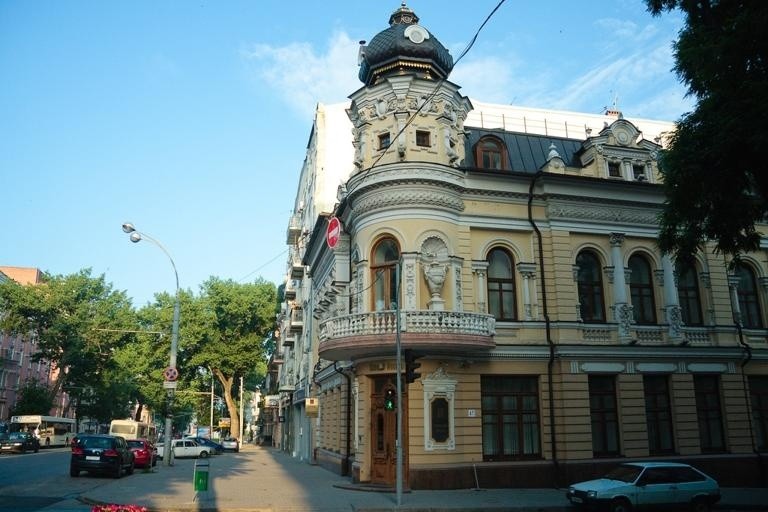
[325,216,340,249]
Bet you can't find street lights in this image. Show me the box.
[119,221,181,466]
[178,345,214,442]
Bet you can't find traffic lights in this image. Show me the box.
[385,387,395,410]
[404,349,422,383]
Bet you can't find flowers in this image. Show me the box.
[90,502,149,512]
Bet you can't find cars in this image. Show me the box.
[125,438,159,470]
[0,432,40,454]
[566,460,723,512]
[149,430,239,459]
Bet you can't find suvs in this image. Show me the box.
[70,433,135,479]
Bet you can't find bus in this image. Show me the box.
[8,415,78,449]
[108,417,158,445]
